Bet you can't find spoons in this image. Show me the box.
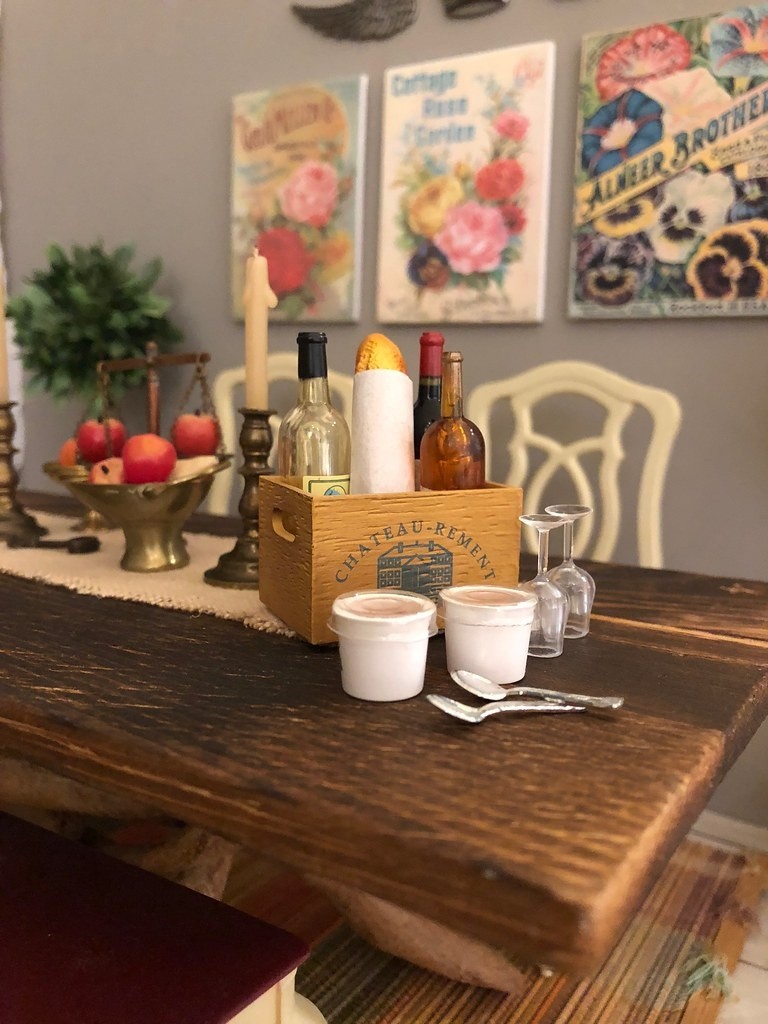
[424,694,587,722]
[450,669,625,711]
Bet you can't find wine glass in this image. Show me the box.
[513,513,571,658]
[544,505,596,640]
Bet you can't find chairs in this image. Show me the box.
[467,360,682,570]
[206,352,354,515]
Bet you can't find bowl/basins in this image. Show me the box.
[42,452,233,572]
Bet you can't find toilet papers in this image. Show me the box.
[350,369,417,495]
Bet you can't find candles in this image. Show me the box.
[241,248,278,409]
[0,243,9,399]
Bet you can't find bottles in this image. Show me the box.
[278,330,351,497]
[418,350,485,491]
[411,330,447,491]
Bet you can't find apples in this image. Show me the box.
[171,407,220,458]
[120,433,177,484]
[59,436,91,469]
[76,416,128,462]
[89,457,125,484]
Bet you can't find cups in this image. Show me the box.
[439,585,540,686]
[327,588,438,703]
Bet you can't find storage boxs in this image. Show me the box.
[261,477,524,645]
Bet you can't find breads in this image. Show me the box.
[354,333,407,376]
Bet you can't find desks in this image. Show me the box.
[0,490,768,992]
